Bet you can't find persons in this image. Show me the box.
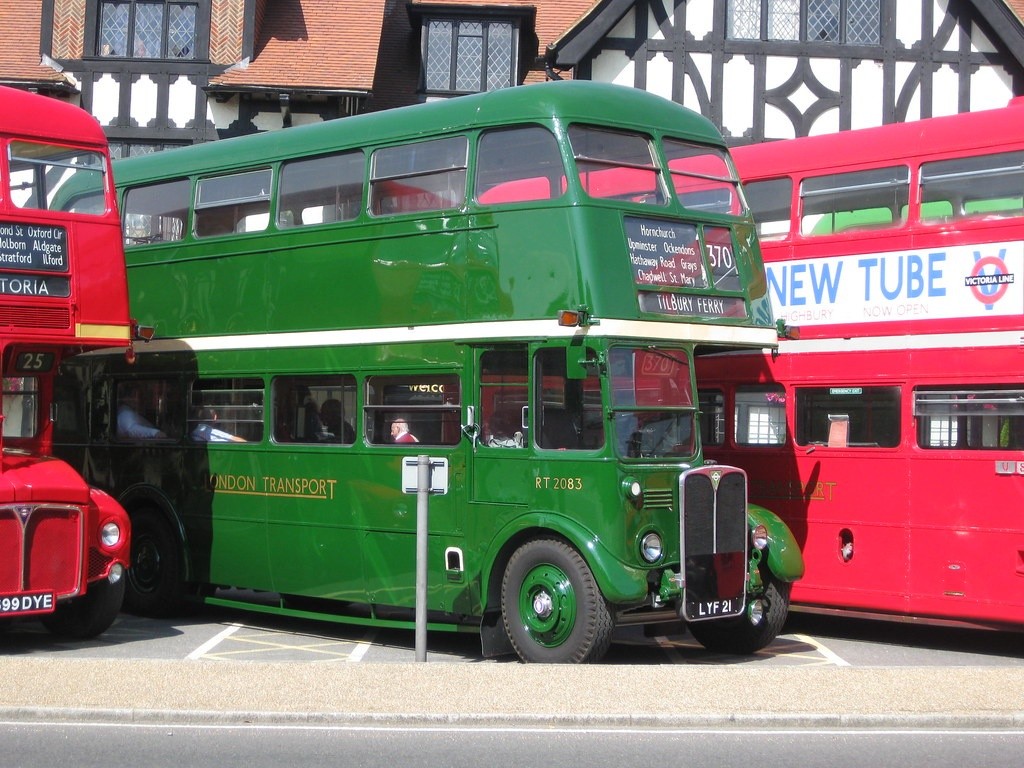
[190,404,248,444]
[115,386,168,439]
[484,410,523,449]
[391,419,419,444]
[276,399,355,444]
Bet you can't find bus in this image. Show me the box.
[33,80,809,669]
[433,94,1024,646]
[0,81,128,647]
